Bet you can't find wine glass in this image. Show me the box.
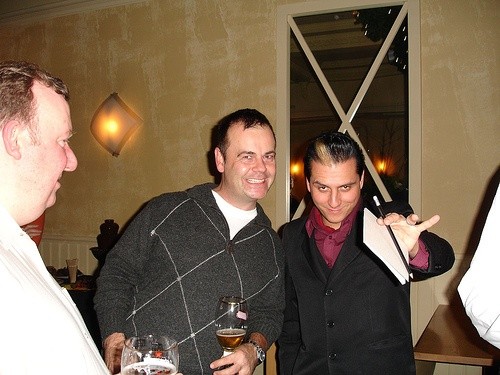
[214,297,249,375]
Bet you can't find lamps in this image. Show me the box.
[89,93,143,157]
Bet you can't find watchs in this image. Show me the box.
[248,340,265,365]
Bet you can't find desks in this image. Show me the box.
[412,303,500,375]
[61,283,104,359]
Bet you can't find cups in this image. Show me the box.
[121,334,179,375]
[66,259,78,289]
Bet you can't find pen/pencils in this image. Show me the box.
[372,195,415,279]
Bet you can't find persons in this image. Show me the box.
[94,108,285,375]
[457,183,500,349]
[0,60,112,375]
[275,131,456,375]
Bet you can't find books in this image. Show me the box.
[363,208,410,285]
